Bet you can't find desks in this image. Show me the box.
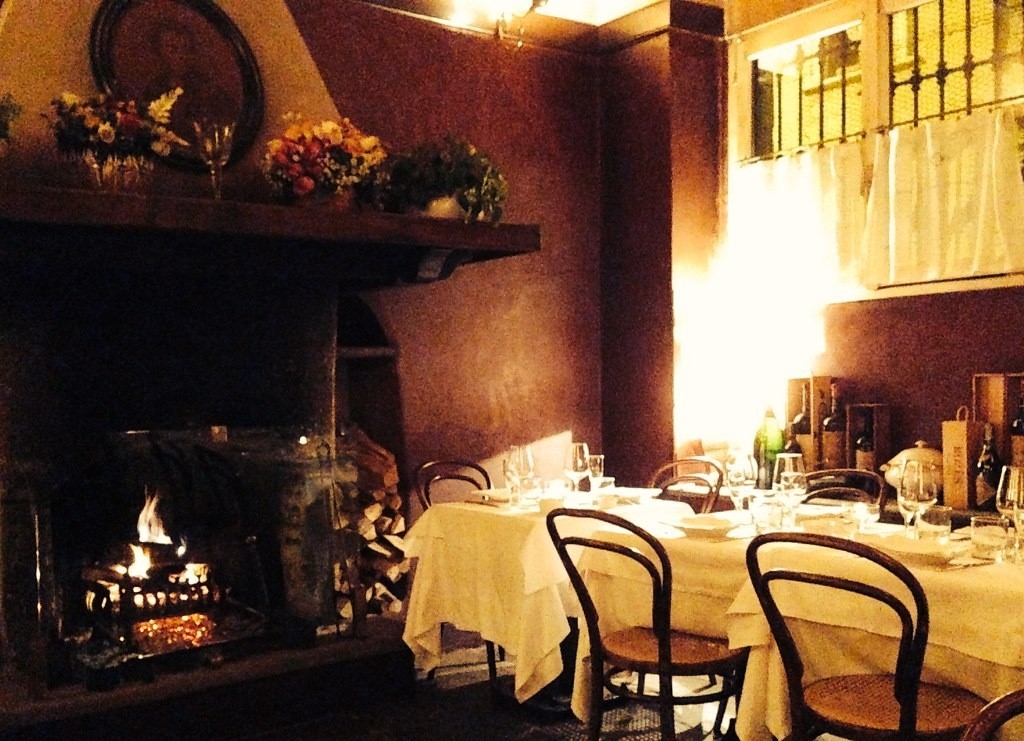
[400,496,697,719]
[570,519,1024,741]
[668,484,1012,526]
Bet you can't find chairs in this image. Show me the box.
[647,455,723,514]
[546,507,751,741]
[745,531,990,741]
[803,468,887,509]
[415,458,506,679]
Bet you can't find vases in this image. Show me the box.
[70,150,158,194]
[293,188,357,213]
[422,194,461,218]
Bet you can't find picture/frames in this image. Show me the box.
[92,0,265,173]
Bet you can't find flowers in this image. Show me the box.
[260,109,392,204]
[384,135,508,229]
[41,87,190,177]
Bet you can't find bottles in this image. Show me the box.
[752,404,781,491]
[821,379,845,472]
[783,421,802,456]
[794,381,812,470]
[973,424,999,512]
[855,414,874,473]
[1009,378,1024,466]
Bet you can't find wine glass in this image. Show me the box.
[772,454,807,534]
[588,455,605,495]
[897,459,937,541]
[501,460,518,506]
[563,443,589,499]
[507,444,534,512]
[896,477,915,538]
[722,452,756,526]
[193,116,237,200]
[996,466,1024,565]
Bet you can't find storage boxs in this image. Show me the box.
[845,402,890,498]
[972,372,1024,465]
[785,374,830,472]
[941,406,986,509]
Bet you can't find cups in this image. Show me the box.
[920,507,951,542]
[602,477,615,488]
[755,505,782,537]
[971,517,1009,565]
[521,475,542,508]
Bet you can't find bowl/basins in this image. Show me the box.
[800,516,859,544]
[660,514,738,537]
